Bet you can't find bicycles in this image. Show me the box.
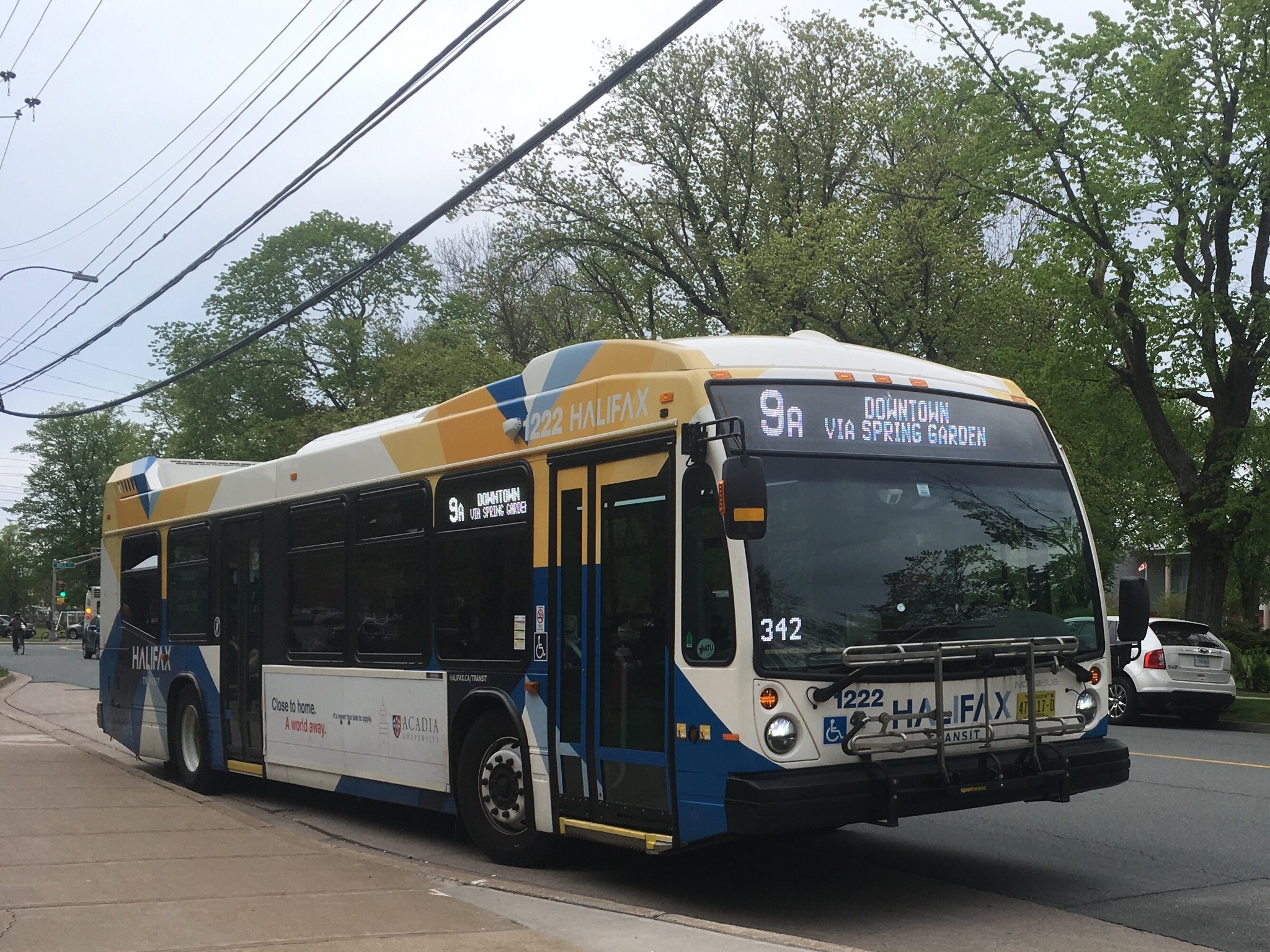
[11,632,26,655]
[41,625,68,642]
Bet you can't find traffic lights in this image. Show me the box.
[60,583,66,597]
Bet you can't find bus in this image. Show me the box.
[92,329,1148,866]
[66,612,85,639]
[83,585,101,631]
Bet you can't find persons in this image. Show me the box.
[8,611,29,651]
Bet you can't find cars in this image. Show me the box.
[1106,614,1237,725]
[82,615,100,660]
[0,614,36,639]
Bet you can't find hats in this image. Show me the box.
[15,611,20,615]
